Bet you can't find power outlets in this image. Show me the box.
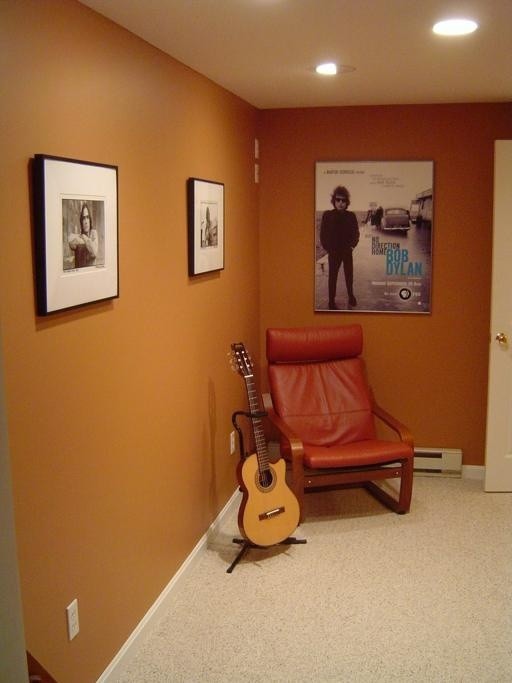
[65,596,80,641]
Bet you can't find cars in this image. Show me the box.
[381,205,412,233]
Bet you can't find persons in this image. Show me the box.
[68,202,99,268]
[204,206,211,247]
[320,185,424,310]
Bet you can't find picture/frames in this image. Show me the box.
[34,153,120,317]
[185,176,226,278]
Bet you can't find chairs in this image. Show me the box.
[260,322,414,523]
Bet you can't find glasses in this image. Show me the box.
[334,197,347,203]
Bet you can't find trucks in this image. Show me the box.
[415,187,432,229]
[409,199,419,222]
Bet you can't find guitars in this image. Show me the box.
[227,343,300,549]
